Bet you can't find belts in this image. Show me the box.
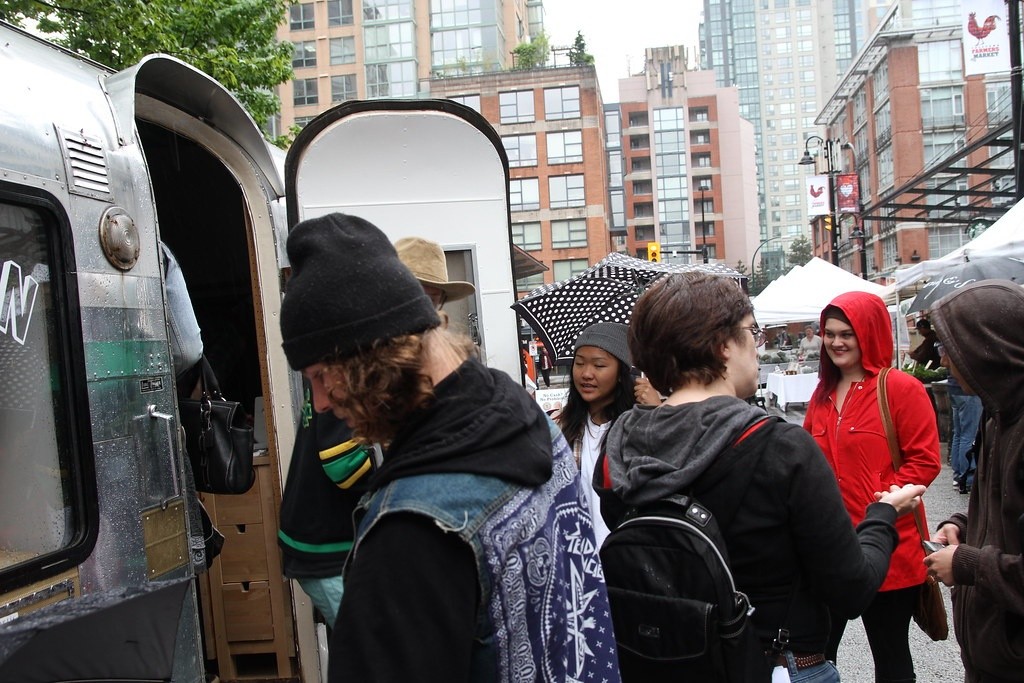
[776,653,825,669]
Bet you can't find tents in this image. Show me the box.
[751,196,1024,371]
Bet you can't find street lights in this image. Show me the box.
[698,185,710,263]
[798,136,841,267]
[751,235,790,294]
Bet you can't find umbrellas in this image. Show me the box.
[906,255,1024,316]
[510,251,760,377]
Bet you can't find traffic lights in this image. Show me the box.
[647,242,661,264]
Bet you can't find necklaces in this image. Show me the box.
[860,375,865,382]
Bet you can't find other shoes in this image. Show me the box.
[953,481,959,490]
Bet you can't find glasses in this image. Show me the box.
[938,346,947,358]
[734,326,768,348]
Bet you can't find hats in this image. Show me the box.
[913,319,930,330]
[392,237,476,304]
[574,323,634,374]
[279,212,442,372]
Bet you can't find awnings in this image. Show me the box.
[513,243,550,279]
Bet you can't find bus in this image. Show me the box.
[0,20,527,683]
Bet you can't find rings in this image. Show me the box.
[928,575,935,584]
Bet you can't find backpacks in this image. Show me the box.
[597,492,802,683]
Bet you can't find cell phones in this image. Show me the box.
[922,540,948,552]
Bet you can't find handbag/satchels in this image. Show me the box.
[176,353,256,496]
[913,571,949,642]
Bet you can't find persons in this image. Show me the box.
[799,325,822,362]
[546,322,662,556]
[280,214,621,683]
[539,347,553,390]
[798,296,936,683]
[161,237,220,683]
[908,279,1024,683]
[782,330,793,349]
[281,238,475,629]
[606,270,928,683]
